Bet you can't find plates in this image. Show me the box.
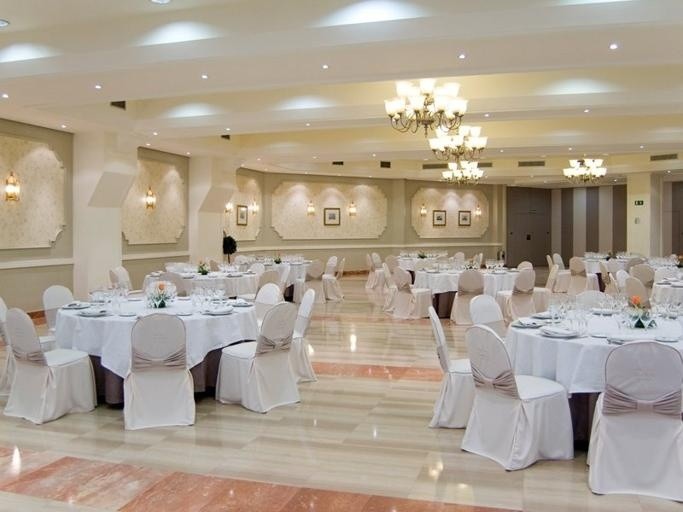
[531,314,559,319]
[509,321,541,328]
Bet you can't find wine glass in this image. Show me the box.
[542,291,683,343]
[62,281,253,318]
[585,251,682,288]
[150,253,303,278]
[399,248,519,274]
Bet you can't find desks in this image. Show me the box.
[506,308,682,452]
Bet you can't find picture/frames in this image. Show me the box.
[324,208,341,226]
[237,204,248,226]
[458,211,471,226]
[432,210,447,227]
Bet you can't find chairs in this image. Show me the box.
[361,246,682,326]
[469,295,508,344]
[461,323,573,472]
[1,249,324,430]
[586,340,681,500]
[428,306,476,430]
[322,256,338,277]
[322,258,346,300]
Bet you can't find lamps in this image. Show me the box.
[142,187,155,208]
[5,170,19,201]
[562,158,608,187]
[442,158,483,187]
[385,77,469,135]
[428,124,488,164]
